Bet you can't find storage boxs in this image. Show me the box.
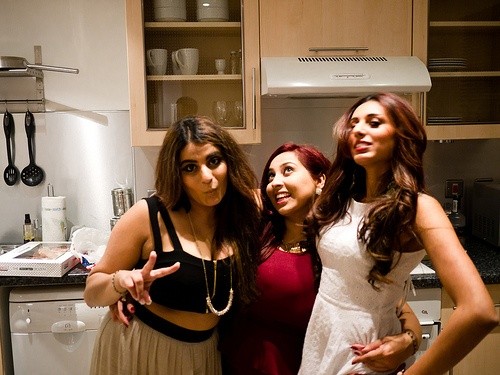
[0,242,81,278]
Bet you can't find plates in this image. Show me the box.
[428,57,469,70]
[427,116,465,122]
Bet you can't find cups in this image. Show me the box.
[145,49,167,75]
[148,103,177,127]
[232,100,243,126]
[197,0,229,22]
[213,100,232,125]
[171,48,199,74]
[154,0,187,22]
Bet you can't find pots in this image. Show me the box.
[0,56,79,74]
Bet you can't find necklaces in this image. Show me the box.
[281,240,300,252]
[188,212,234,316]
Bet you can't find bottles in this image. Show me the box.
[110,217,120,230]
[229,48,242,74]
[23,214,35,243]
[449,183,466,246]
[111,188,135,217]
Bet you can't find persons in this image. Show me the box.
[300,93,500,375]
[109,144,422,375]
[83,117,263,375]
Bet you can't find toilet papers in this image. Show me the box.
[41,195,67,242]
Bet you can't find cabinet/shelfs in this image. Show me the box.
[412,0,500,140]
[441,283,500,375]
[125,0,261,146]
[258,0,413,57]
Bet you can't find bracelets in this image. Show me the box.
[404,329,419,356]
[112,270,128,294]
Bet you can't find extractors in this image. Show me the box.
[261,56,432,99]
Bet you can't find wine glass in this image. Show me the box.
[215,59,226,75]
